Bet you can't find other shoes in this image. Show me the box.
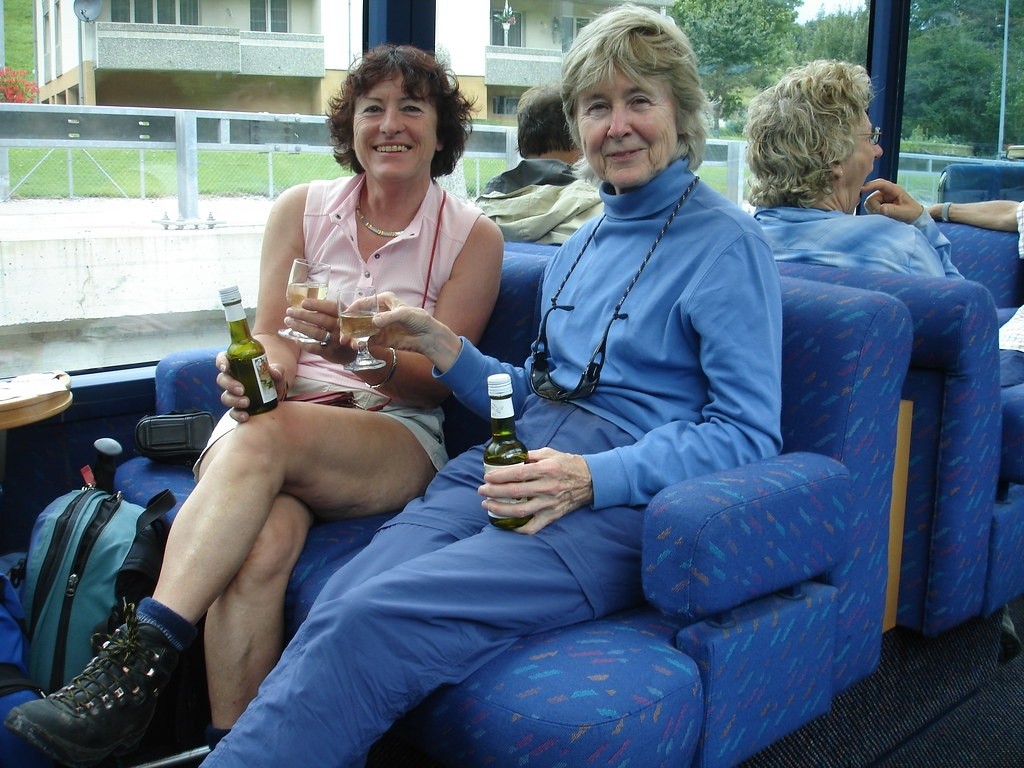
[998,604,1023,663]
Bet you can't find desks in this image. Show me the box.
[1,370,73,483]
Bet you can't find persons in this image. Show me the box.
[4,44,503,768]
[199,7,784,768]
[470,80,606,246]
[743,60,1020,664]
[928,200,1024,386]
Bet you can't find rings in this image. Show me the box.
[320,332,331,345]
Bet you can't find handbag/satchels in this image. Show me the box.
[135,410,215,466]
[0,573,53,768]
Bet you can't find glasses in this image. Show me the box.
[530,306,628,400]
[856,126,882,145]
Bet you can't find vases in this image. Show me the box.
[502,22,511,31]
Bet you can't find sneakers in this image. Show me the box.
[4,597,179,768]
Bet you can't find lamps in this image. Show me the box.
[552,16,560,44]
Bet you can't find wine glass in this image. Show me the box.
[338,287,386,372]
[278,259,333,344]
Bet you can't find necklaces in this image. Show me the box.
[355,191,404,237]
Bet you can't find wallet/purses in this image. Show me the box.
[284,391,354,406]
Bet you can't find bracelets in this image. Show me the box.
[941,203,951,222]
[365,347,398,389]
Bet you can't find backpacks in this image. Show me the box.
[0,464,176,697]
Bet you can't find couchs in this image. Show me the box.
[115,252,912,768]
[776,261,1024,638]
[931,221,1024,486]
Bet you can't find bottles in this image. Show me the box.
[484,373,530,530]
[219,285,279,416]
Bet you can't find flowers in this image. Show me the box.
[494,6,516,30]
